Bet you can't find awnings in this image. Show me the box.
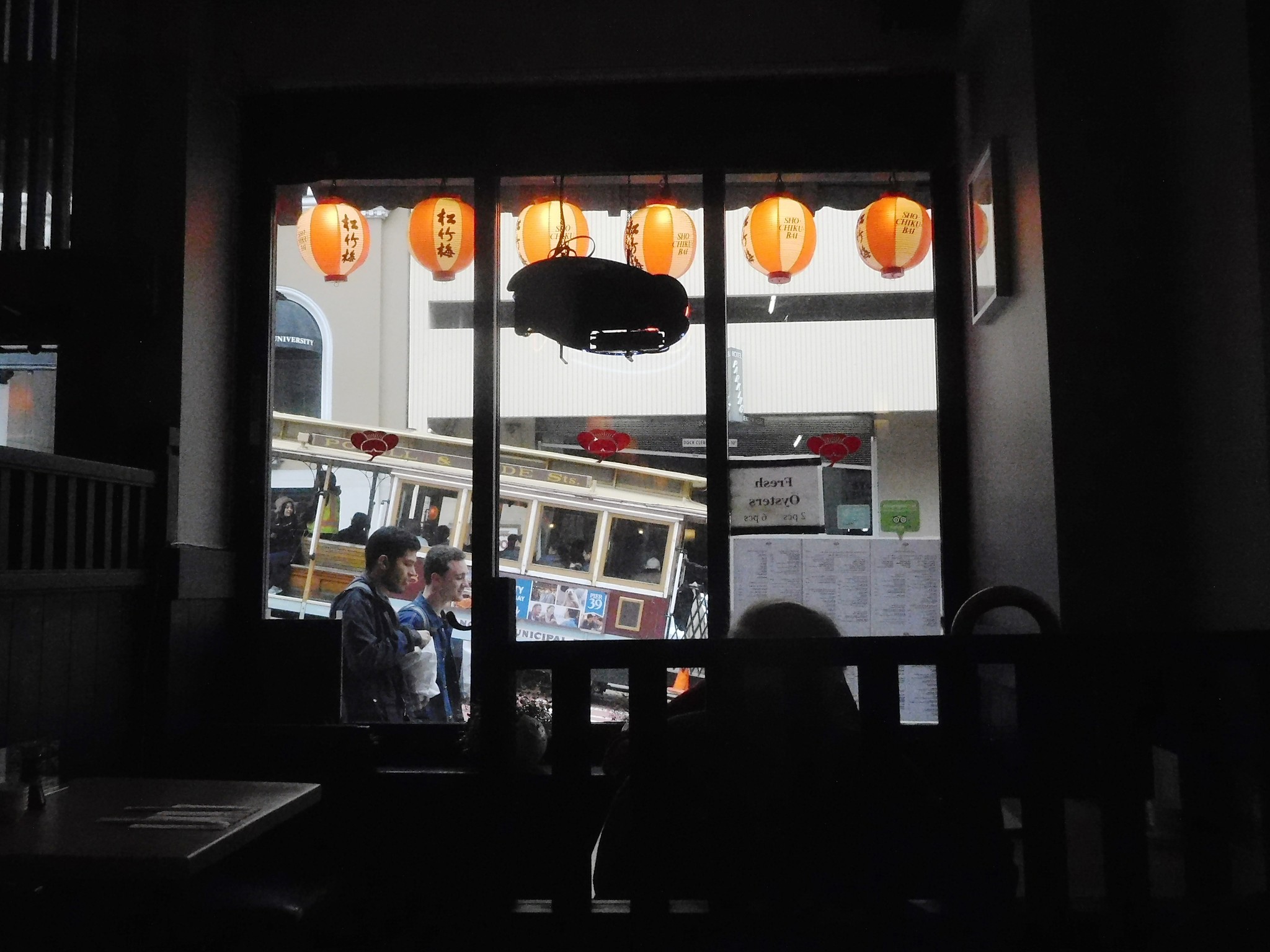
[274,300,322,358]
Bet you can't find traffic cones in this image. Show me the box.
[667,667,690,694]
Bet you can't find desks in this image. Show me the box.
[0,774,327,952]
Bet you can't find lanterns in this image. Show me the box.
[855,191,932,281]
[973,201,988,260]
[297,196,370,287]
[741,193,817,286]
[407,193,474,284]
[623,198,696,279]
[515,195,589,267]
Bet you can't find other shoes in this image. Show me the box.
[268,586,282,594]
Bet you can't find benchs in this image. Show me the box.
[286,536,368,605]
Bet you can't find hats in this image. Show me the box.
[274,496,296,515]
[645,558,661,570]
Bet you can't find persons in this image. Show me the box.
[337,512,370,545]
[629,557,662,585]
[404,519,428,546]
[438,525,450,545]
[500,533,561,568]
[300,470,342,540]
[463,533,472,553]
[528,587,603,632]
[422,519,441,546]
[594,603,862,899]
[341,525,468,724]
[574,547,592,572]
[268,496,303,595]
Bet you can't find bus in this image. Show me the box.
[271,409,707,699]
